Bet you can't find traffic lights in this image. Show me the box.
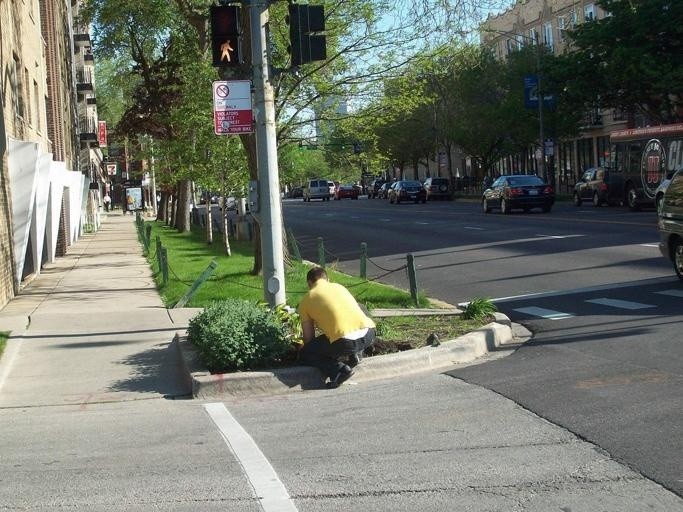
[289,3,327,64]
[211,5,243,66]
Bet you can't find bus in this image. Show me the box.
[607,123,682,212]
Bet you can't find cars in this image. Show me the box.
[200,191,246,210]
[292,180,360,202]
[573,168,603,207]
[368,178,451,204]
[482,175,554,214]
[654,167,683,278]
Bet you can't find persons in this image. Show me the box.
[300,266,376,389]
[103,192,110,212]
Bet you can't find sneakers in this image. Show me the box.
[327,365,355,389]
[349,352,363,366]
